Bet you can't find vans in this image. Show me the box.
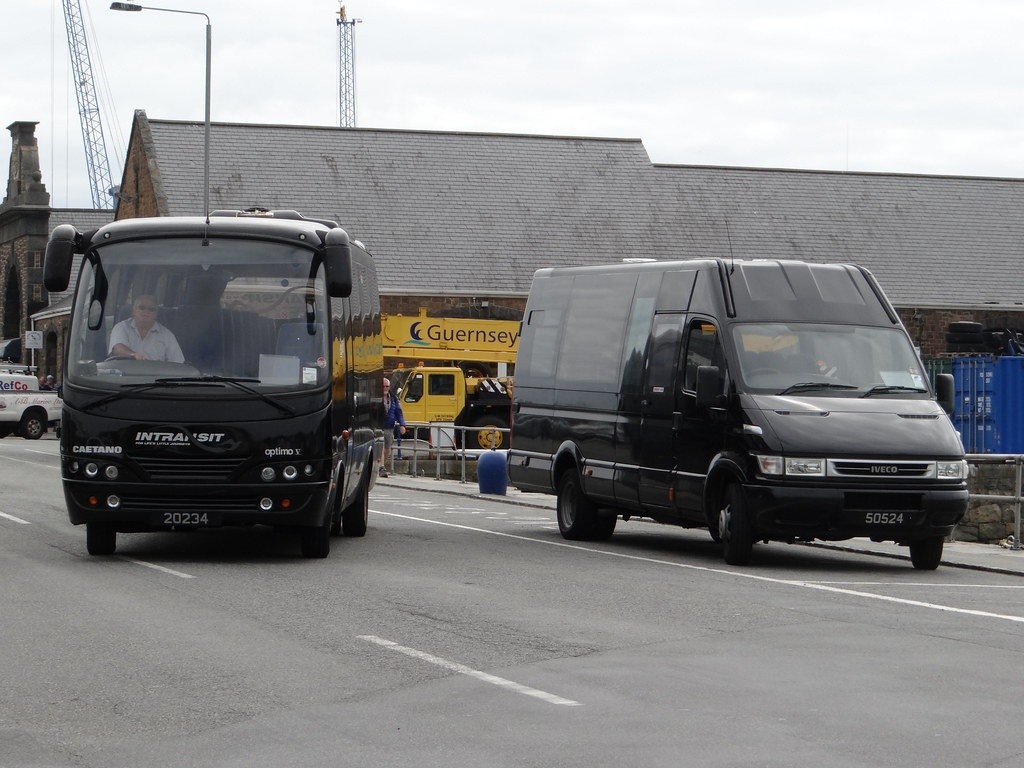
[503,256,971,570]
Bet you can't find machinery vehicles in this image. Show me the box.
[45,1,127,209]
[336,1,363,127]
[379,304,521,449]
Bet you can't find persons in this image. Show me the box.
[378,378,406,477]
[39,374,60,391]
[108,294,185,364]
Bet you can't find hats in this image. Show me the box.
[47,375,54,380]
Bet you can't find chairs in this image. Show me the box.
[162,304,326,379]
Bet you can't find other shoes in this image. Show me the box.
[379,466,388,476]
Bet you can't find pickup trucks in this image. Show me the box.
[0,364,64,441]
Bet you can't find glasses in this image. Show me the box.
[383,386,390,388]
[133,305,157,312]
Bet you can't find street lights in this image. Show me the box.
[109,0,214,216]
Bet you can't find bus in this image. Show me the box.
[38,205,389,558]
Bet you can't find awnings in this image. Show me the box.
[0,337,22,359]
